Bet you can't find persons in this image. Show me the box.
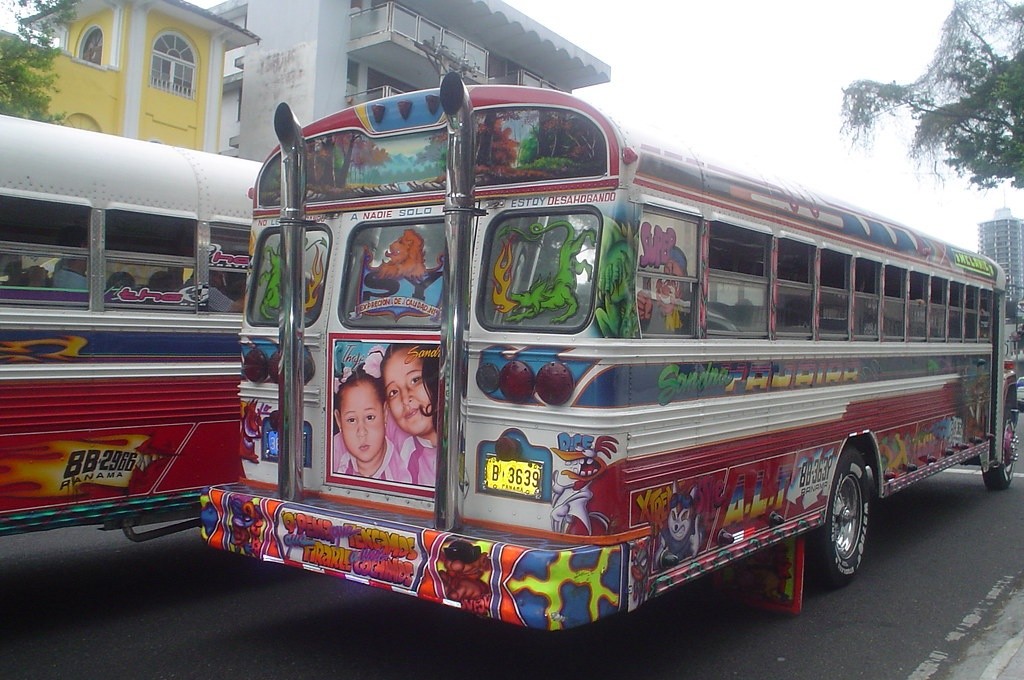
[4,258,246,315]
[333,343,437,490]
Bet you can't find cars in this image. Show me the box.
[1016,376,1024,403]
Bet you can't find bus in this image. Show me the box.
[199,70,1019,635]
[1,114,302,543]
